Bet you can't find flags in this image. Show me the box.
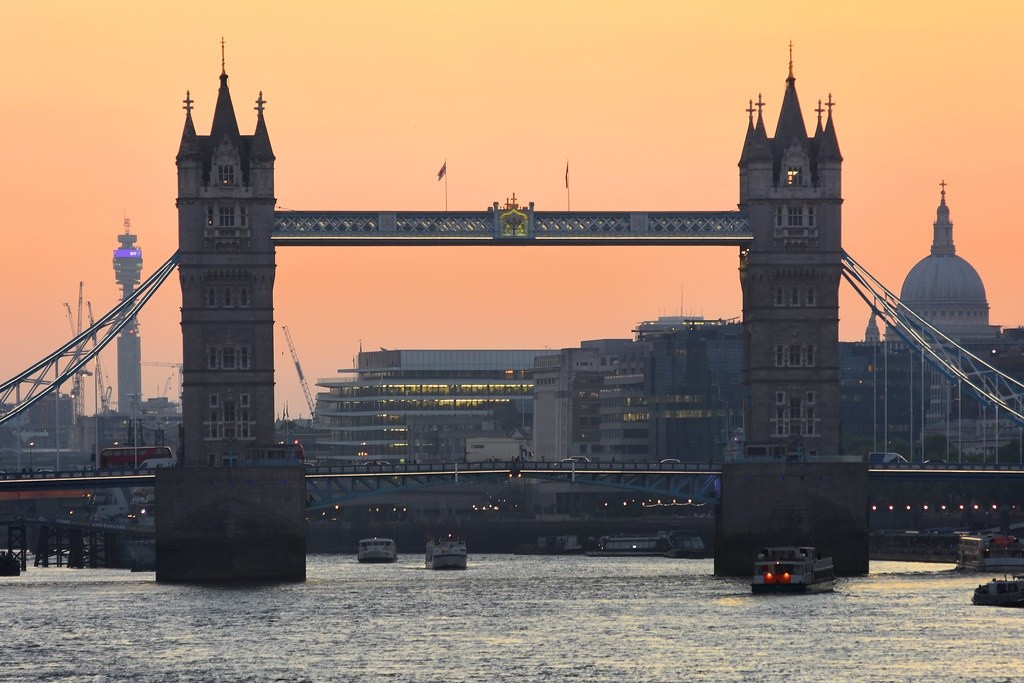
[437,162,446,183]
[566,163,568,188]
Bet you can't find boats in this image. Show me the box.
[958,533,1024,575]
[425,533,468,571]
[971,575,1024,607]
[750,545,835,593]
[0,551,21,578]
[359,538,398,563]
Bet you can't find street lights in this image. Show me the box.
[29,442,33,473]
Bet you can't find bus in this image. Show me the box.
[100,447,175,475]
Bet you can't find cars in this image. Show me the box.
[653,459,681,469]
[560,456,590,470]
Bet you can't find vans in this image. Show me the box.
[868,451,910,470]
